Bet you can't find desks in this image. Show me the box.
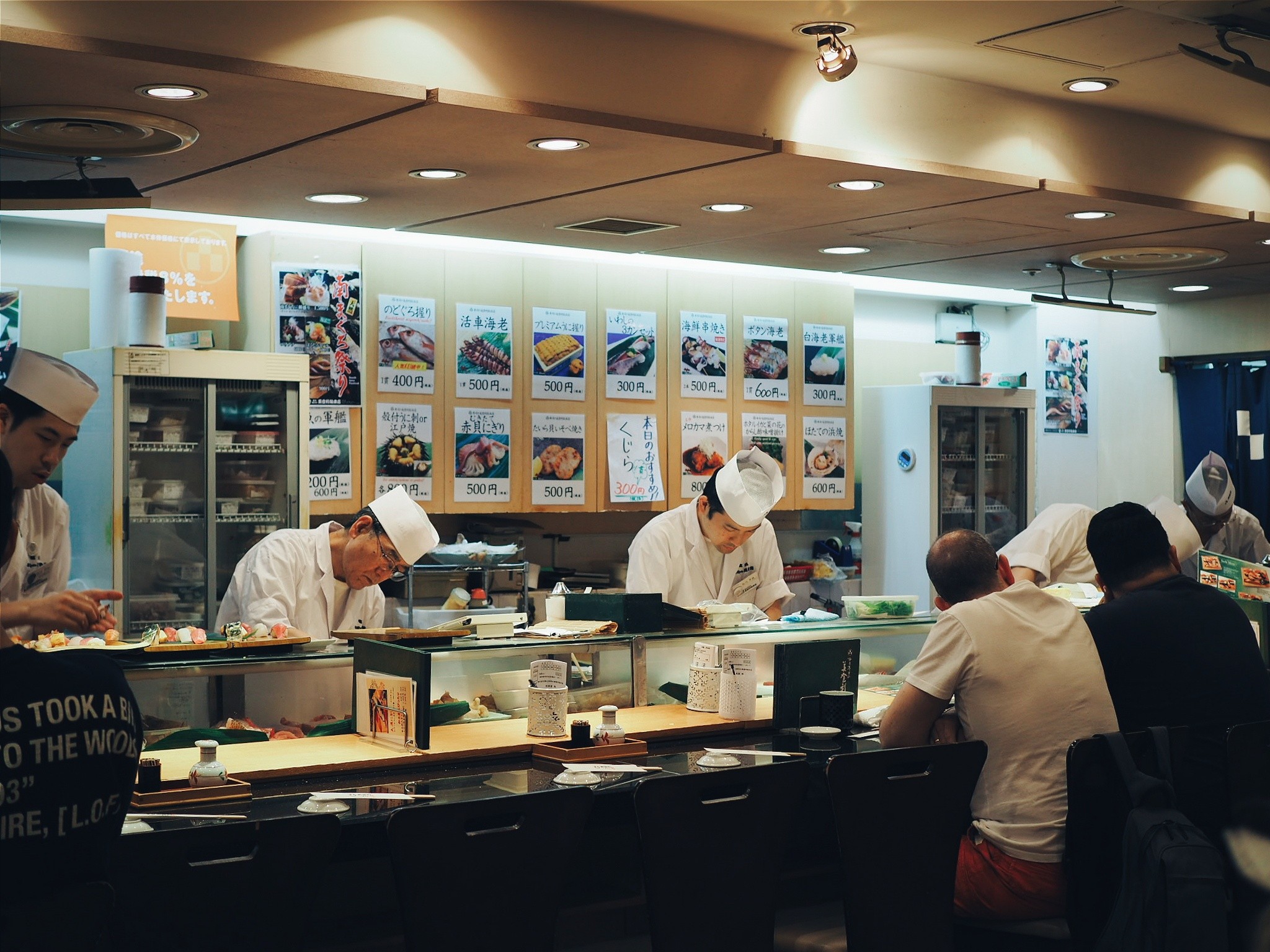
[124,732,992,952]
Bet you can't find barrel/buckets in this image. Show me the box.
[128,276,166,349]
[955,332,981,385]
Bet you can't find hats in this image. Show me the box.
[715,445,784,527]
[4,348,100,427]
[1146,496,1204,563]
[367,485,440,565]
[1186,450,1236,515]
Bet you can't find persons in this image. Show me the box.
[996,497,1205,586]
[215,485,439,637]
[1082,502,1269,754]
[1176,450,1269,564]
[625,446,796,622]
[1,346,124,649]
[879,530,1121,919]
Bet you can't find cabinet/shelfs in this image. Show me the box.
[353,613,946,751]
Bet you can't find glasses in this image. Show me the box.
[365,512,409,581]
[1185,499,1236,529]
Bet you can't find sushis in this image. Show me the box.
[11,622,336,754]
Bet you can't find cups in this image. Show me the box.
[820,690,853,731]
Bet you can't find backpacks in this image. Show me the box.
[1084,725,1231,952]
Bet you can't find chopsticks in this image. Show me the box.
[126,814,247,819]
[786,753,807,756]
[639,767,662,771]
[409,794,436,798]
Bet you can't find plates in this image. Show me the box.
[297,796,350,813]
[441,712,511,725]
[553,770,601,785]
[800,726,841,739]
[696,752,741,767]
[293,638,335,653]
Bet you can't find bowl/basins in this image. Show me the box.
[484,667,531,718]
[841,595,919,620]
[121,819,154,834]
[127,402,278,633]
